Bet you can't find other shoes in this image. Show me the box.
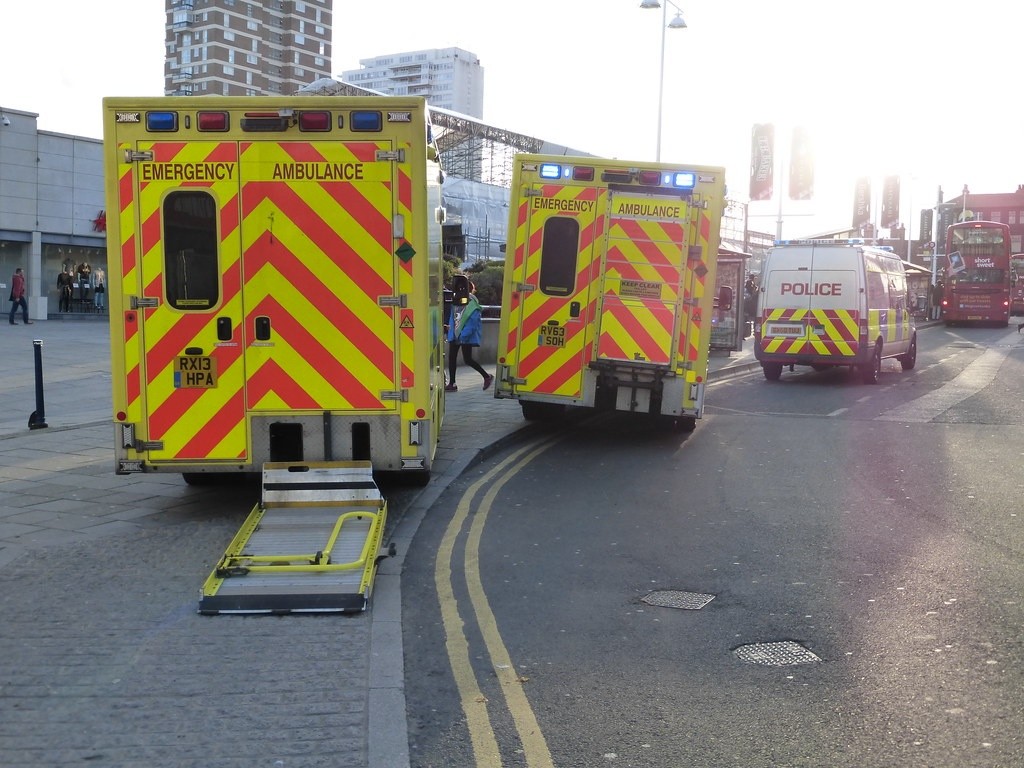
[10,322,19,325]
[25,321,33,324]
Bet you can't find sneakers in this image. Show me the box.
[445,383,458,392]
[483,374,494,390]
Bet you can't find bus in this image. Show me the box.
[942,221,1010,327]
[1010,255,1024,318]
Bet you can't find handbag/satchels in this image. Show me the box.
[8,287,15,301]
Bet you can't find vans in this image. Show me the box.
[754,237,917,384]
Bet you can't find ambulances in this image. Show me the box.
[493,154,733,433]
[103,96,470,614]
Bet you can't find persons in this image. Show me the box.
[445,280,493,392]
[928,279,944,321]
[56,258,107,313]
[9,268,35,325]
[745,274,760,296]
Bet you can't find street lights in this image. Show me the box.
[639,0,687,163]
